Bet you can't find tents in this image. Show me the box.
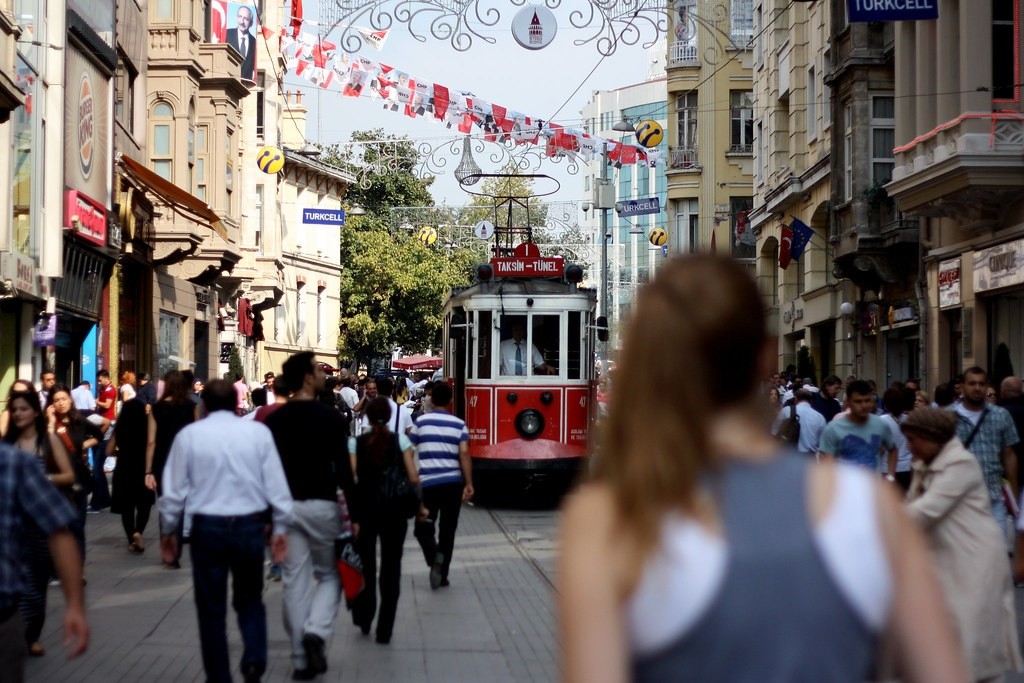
[393,354,444,370]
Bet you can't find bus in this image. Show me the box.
[430,174,614,502]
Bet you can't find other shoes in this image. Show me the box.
[301,631,327,675]
[242,674,261,683]
[29,645,44,657]
[292,668,316,680]
[441,579,449,588]
[430,553,444,590]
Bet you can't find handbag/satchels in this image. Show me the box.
[387,468,420,521]
[776,404,800,448]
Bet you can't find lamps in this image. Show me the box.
[121,241,133,253]
[64,215,82,232]
[611,116,635,131]
[840,302,854,317]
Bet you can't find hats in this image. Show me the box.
[905,407,958,440]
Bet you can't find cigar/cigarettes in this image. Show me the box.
[466,501,474,506]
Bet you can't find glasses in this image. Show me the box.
[986,392,997,398]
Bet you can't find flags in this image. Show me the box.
[279,0,658,168]
[778,218,815,270]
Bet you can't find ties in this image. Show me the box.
[241,37,246,57]
[514,343,522,375]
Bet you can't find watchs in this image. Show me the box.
[886,475,896,482]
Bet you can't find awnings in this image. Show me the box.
[115,152,229,244]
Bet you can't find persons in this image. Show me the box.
[263,351,365,681]
[559,251,971,683]
[160,379,297,683]
[995,376,1024,499]
[0,369,443,570]
[0,443,90,683]
[500,322,557,375]
[767,363,997,483]
[596,381,609,415]
[408,380,474,591]
[897,407,1024,683]
[348,397,420,645]
[944,365,1020,547]
[226,6,256,80]
